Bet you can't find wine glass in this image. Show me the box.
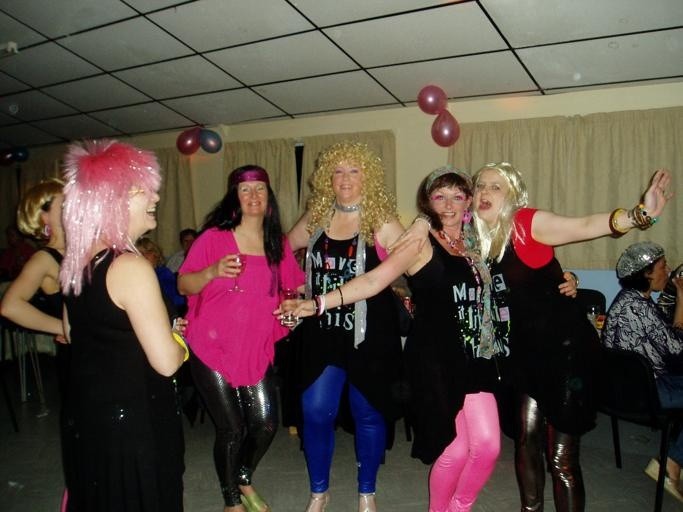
[224,253,247,292]
[588,304,600,320]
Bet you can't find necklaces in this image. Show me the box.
[438,228,493,314]
[330,199,360,214]
[321,204,361,287]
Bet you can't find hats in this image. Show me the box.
[616,241,663,278]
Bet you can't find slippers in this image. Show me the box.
[240,493,271,512]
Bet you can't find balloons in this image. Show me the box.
[416,84,448,115]
[176,125,201,155]
[430,109,460,149]
[198,128,221,154]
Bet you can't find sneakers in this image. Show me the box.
[644,458,683,500]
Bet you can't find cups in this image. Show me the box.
[276,291,298,326]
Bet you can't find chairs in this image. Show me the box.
[574,290,605,316]
[602,348,682,512]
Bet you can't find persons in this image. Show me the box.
[163,228,197,273]
[0,177,74,511]
[175,164,306,512]
[387,158,677,511]
[657,260,682,332]
[599,240,682,504]
[271,163,580,512]
[284,139,411,512]
[136,236,188,328]
[58,136,191,510]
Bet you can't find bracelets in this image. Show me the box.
[313,290,327,319]
[627,203,662,232]
[172,332,189,363]
[569,271,579,287]
[608,205,630,236]
[335,285,346,310]
[412,214,433,230]
